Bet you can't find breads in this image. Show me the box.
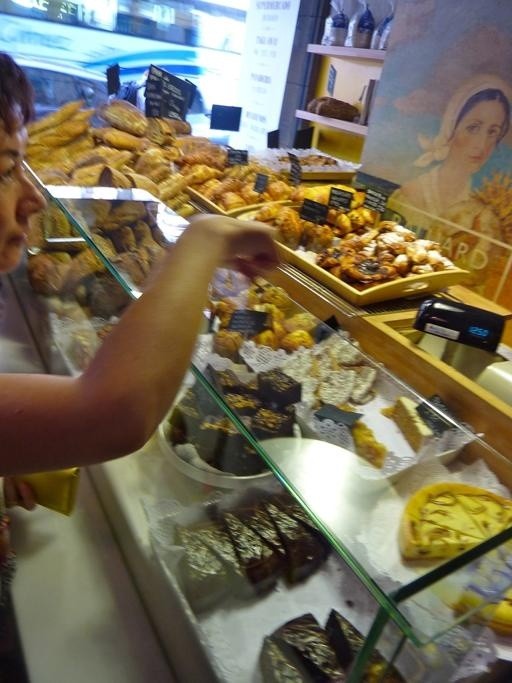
[176,497,331,615]
[260,609,410,683]
[24,201,321,373]
[281,330,434,468]
[25,98,298,219]
[253,184,455,292]
[171,368,303,477]
[307,98,360,122]
[398,482,512,637]
[278,154,337,167]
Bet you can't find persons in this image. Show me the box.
[387,73,511,242]
[2,49,280,683]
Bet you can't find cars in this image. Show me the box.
[16,57,142,126]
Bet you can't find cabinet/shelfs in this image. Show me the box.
[290,36,388,137]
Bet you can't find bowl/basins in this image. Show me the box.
[156,410,305,492]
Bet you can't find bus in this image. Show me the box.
[0,1,248,81]
